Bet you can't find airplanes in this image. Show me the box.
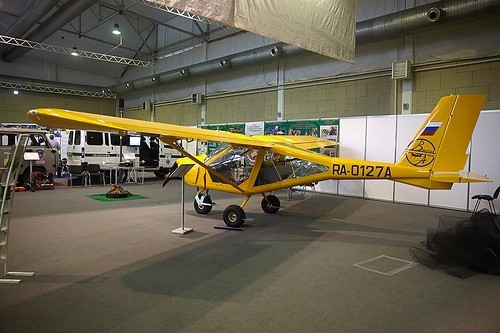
[26,93,494,228]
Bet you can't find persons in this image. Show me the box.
[49,135,61,178]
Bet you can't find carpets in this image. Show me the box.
[87,194,148,202]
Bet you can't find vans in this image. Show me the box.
[0,122,60,187]
[66,129,183,184]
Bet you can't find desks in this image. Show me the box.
[110,167,145,185]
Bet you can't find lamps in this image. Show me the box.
[112,15,120,34]
[71,36,78,56]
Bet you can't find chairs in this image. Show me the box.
[69,164,105,188]
[472,186,500,219]
[140,135,159,157]
